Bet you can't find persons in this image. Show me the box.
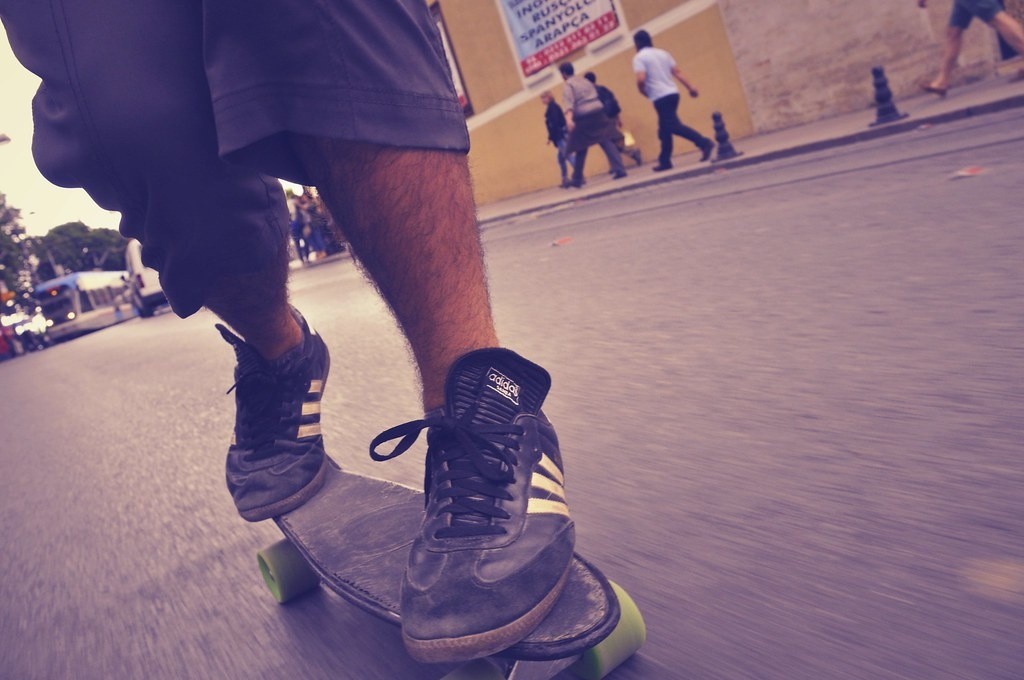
[917,0,1024,95]
[542,62,641,189]
[0,0,576,662]
[633,30,715,171]
[290,193,343,265]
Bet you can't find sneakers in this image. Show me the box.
[215,303,330,523]
[369,348,575,663]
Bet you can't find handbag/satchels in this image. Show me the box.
[620,125,636,150]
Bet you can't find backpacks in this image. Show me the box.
[595,86,621,117]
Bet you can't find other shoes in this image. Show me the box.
[567,180,580,188]
[559,183,568,188]
[919,80,947,98]
[700,140,715,161]
[632,149,641,167]
[653,164,673,171]
[612,173,626,179]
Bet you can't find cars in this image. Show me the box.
[122,238,168,319]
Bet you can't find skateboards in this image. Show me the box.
[256,456,647,680]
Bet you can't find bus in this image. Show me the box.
[34,270,136,341]
